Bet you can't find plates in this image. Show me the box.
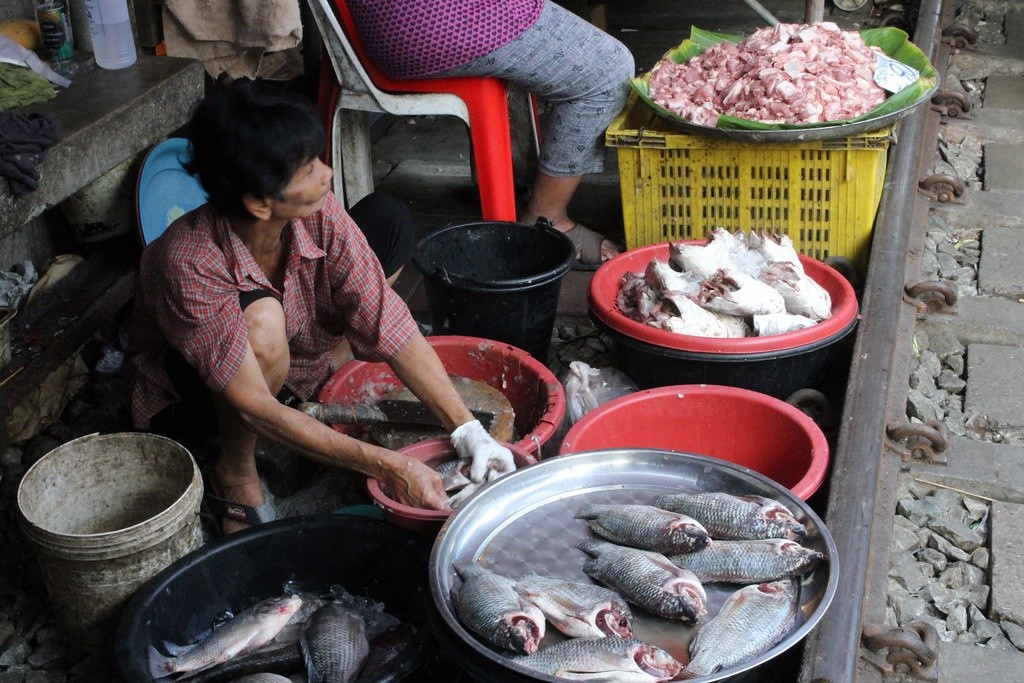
[641,64,942,142]
[429,448,841,683]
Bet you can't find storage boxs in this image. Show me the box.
[605,87,897,268]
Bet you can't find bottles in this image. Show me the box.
[83,0,137,70]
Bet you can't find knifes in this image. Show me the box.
[298,399,494,434]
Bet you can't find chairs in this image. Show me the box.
[306,0,542,219]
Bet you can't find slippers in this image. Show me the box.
[200,443,285,538]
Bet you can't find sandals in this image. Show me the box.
[516,220,624,271]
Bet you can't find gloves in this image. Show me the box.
[449,420,516,482]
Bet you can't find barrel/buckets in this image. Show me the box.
[410,216,579,366]
[15,429,206,645]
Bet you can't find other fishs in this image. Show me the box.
[148,594,426,683]
[449,492,829,683]
[432,456,505,511]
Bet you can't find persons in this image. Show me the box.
[139,77,518,537]
[345,0,635,272]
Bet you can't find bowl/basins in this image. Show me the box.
[558,383,830,502]
[367,436,539,535]
[115,513,438,683]
[586,239,861,399]
[133,136,209,250]
[317,335,567,453]
